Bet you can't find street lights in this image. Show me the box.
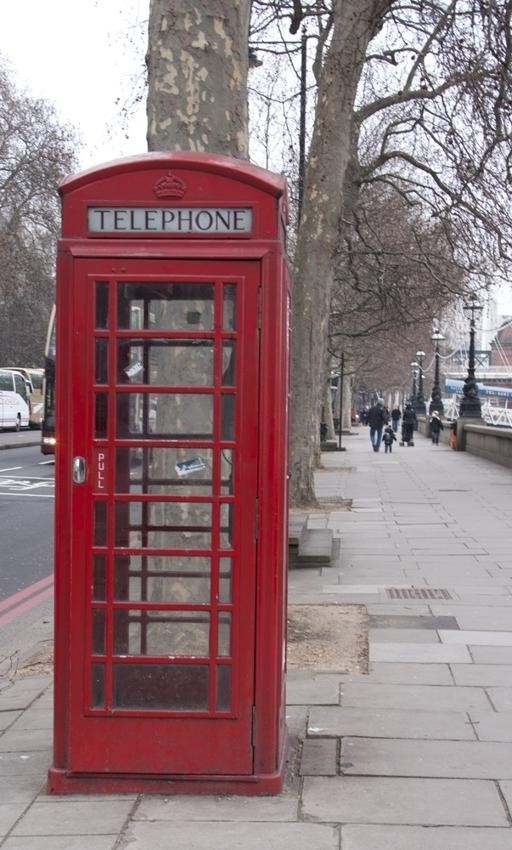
[408,359,418,414]
[429,327,447,418]
[413,348,427,417]
[455,291,487,420]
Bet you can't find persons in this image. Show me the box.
[428,410,443,447]
[351,397,418,454]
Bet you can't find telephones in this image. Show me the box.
[221,348,237,440]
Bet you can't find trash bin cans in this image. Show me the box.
[321,423,329,442]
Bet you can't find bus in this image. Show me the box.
[36,293,160,464]
[0,366,45,432]
